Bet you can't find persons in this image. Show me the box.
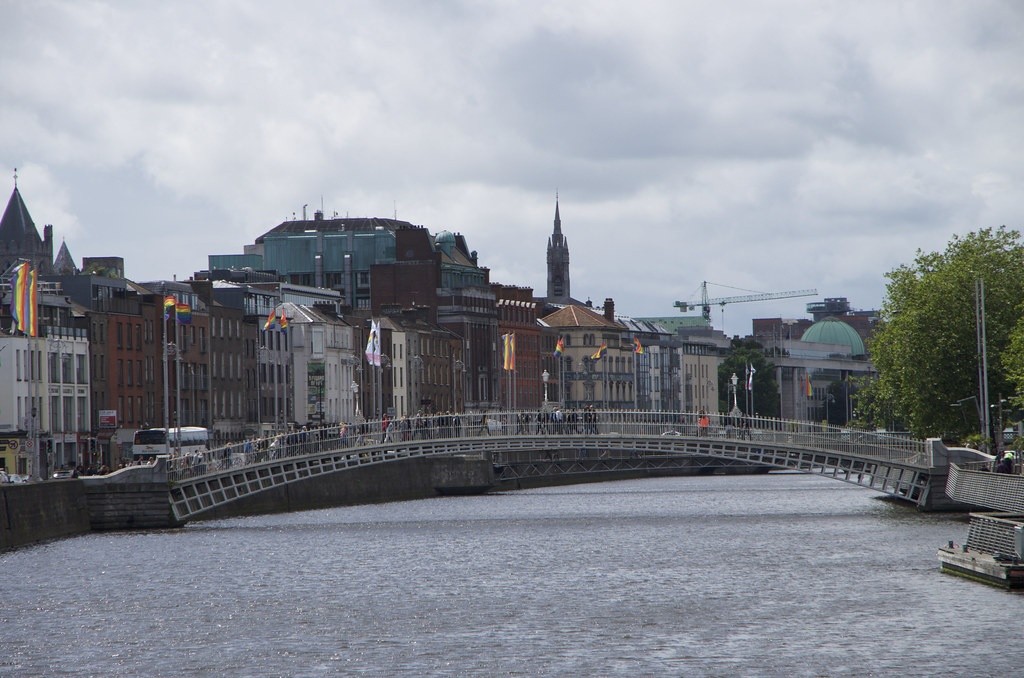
[980,449,1013,477]
[70,404,599,475]
[679,408,752,442]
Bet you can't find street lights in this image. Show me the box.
[731,372,739,406]
[350,381,359,416]
[541,369,551,401]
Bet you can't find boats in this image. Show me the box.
[935,510,1024,591]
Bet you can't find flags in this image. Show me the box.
[633,335,645,355]
[280,309,288,336]
[365,319,381,367]
[175,303,192,325]
[164,296,176,320]
[805,371,814,396]
[262,308,276,333]
[745,363,757,391]
[553,336,565,359]
[501,334,516,371]
[591,341,608,363]
[10,262,37,336]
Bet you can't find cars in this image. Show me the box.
[0,469,79,484]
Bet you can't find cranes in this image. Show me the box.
[674,280,820,324]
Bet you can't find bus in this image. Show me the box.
[132,424,208,465]
